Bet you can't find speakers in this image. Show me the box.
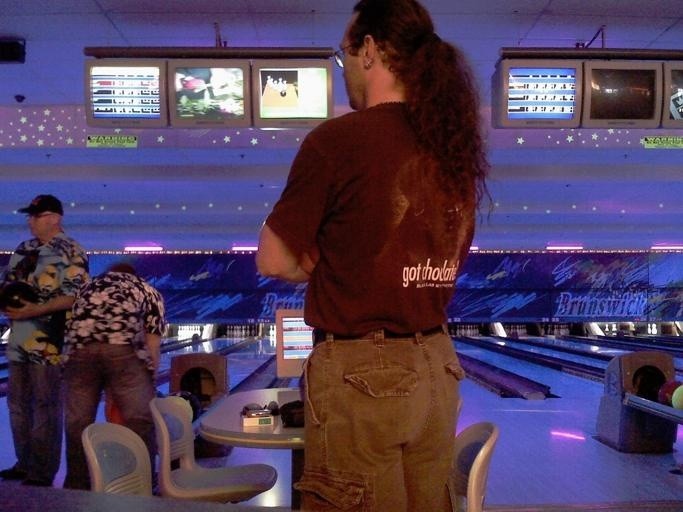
[0,34,26,64]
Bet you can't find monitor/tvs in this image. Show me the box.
[490,59,583,130]
[84,58,168,129]
[251,59,335,129]
[661,60,683,130]
[582,58,663,129]
[275,309,318,378]
[167,58,250,128]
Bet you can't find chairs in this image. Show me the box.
[80,396,279,505]
[446,423,499,512]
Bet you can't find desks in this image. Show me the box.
[199,387,308,512]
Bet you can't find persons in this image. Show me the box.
[62,261,164,496]
[253,2,491,512]
[0,193,90,486]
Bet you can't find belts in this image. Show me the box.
[312,325,442,342]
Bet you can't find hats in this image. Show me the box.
[18,195,63,216]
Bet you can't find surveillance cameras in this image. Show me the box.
[14,95,25,103]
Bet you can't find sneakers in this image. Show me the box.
[0,468,52,486]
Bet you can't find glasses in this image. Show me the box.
[334,45,352,68]
[241,401,279,416]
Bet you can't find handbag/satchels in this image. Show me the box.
[280,400,303,427]
